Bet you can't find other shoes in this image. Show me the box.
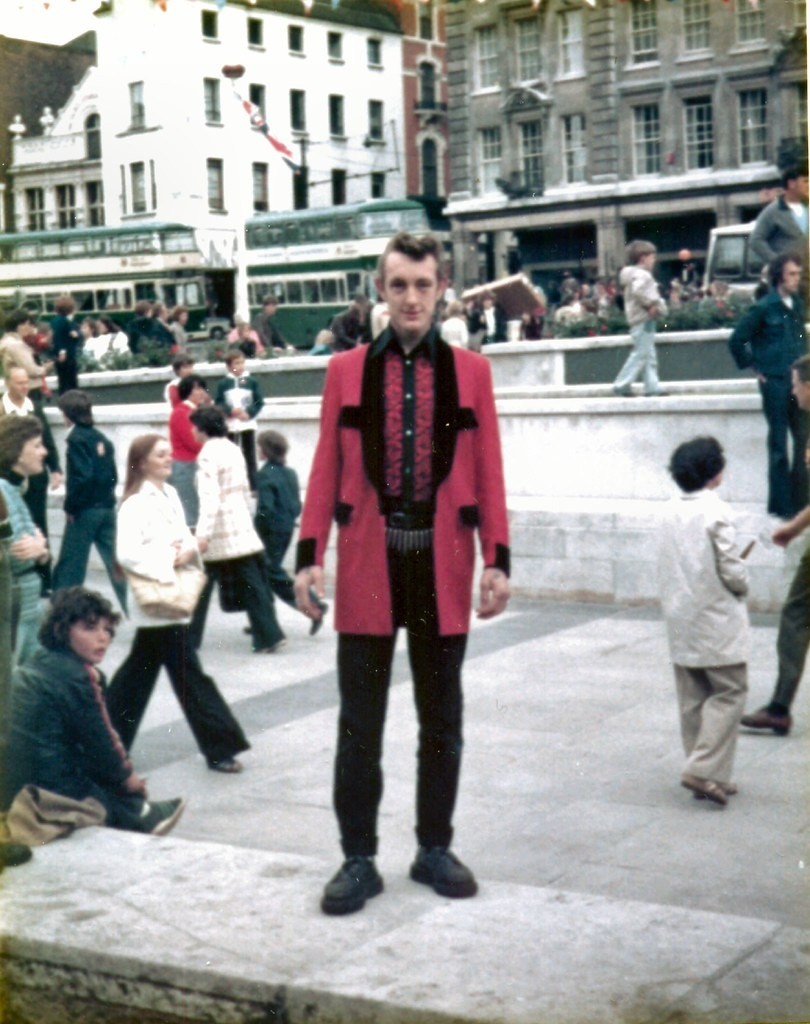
[134,796,186,834]
[321,855,383,914]
[208,757,241,774]
[679,773,739,807]
[647,389,668,396]
[410,846,478,898]
[615,384,638,397]
[310,603,328,634]
[740,706,791,730]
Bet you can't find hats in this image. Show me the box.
[258,431,288,466]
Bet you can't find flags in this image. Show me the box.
[234,91,302,171]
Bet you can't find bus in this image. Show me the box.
[0,222,211,343]
[231,196,437,352]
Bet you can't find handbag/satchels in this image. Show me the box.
[1,783,107,849]
[125,563,208,619]
[216,551,273,613]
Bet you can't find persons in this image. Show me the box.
[741,355,810,736]
[657,438,751,807]
[294,233,511,916]
[612,241,670,396]
[730,250,810,519]
[749,165,809,303]
[309,258,769,354]
[0,291,328,871]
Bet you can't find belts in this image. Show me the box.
[384,514,434,529]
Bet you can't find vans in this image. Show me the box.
[697,223,763,306]
